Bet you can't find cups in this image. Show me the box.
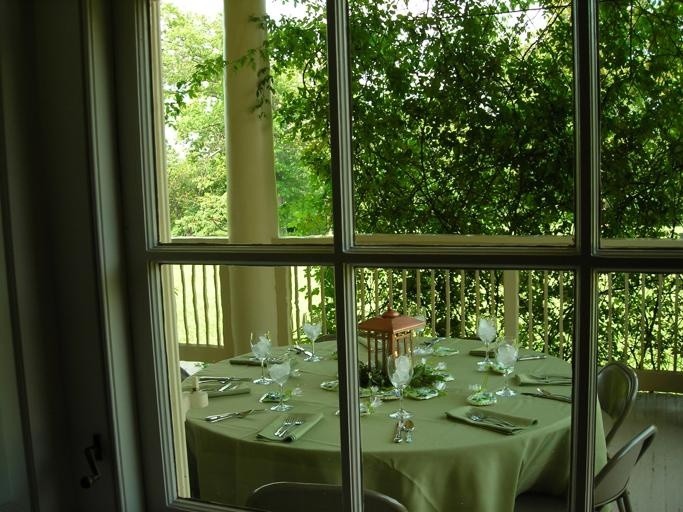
[191,391,208,408]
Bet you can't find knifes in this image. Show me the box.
[294,343,320,356]
[198,375,251,380]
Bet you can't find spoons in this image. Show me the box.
[471,408,515,427]
[536,386,573,402]
[403,419,415,443]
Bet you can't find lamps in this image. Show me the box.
[358,305,426,376]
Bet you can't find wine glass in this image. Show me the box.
[303,310,322,362]
[250,329,290,412]
[388,354,414,420]
[477,315,519,397]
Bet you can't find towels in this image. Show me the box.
[192,383,250,397]
[514,371,571,387]
[469,342,498,358]
[229,355,287,365]
[444,405,540,437]
[255,411,323,443]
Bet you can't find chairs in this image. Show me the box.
[245,482,408,512]
[592,425,659,511]
[596,361,639,446]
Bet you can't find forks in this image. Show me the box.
[211,410,257,423]
[274,416,305,438]
